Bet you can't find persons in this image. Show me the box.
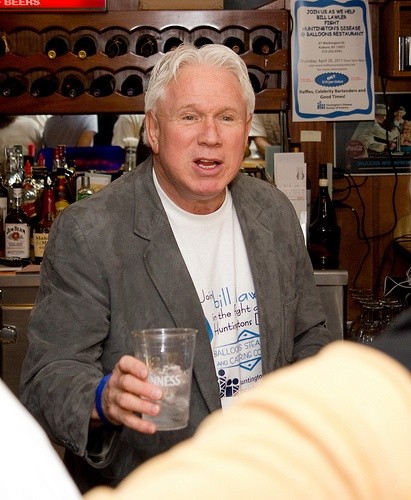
[19,45,333,495]
[0,113,280,165]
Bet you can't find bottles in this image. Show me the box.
[0,37,270,99]
[0,143,96,265]
[307,179,340,271]
[111,137,139,182]
[288,142,314,230]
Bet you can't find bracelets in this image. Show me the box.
[95,373,123,428]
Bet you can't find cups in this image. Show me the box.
[131,327,198,430]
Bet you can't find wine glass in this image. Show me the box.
[350,287,402,346]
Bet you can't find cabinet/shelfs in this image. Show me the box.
[384,0,411,78]
[0,264,349,489]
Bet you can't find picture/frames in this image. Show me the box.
[333,92,411,177]
